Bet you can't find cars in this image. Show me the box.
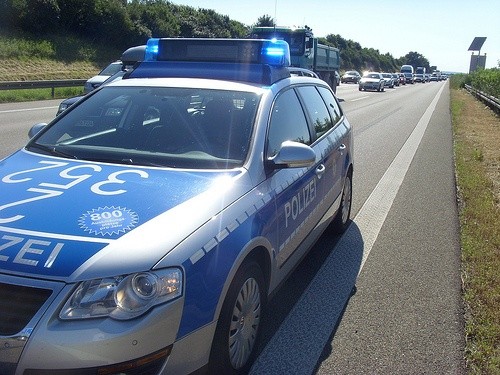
[391,73,400,86]
[55,45,192,139]
[413,74,425,83]
[335,70,340,86]
[341,70,361,84]
[359,72,384,91]
[442,75,447,81]
[380,73,394,88]
[405,73,414,84]
[438,75,442,82]
[85,60,123,95]
[398,73,407,85]
[0,36,355,375]
[425,74,431,83]
[430,75,438,82]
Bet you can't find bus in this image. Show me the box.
[400,65,414,73]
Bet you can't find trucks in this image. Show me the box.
[416,67,426,74]
[432,69,442,76]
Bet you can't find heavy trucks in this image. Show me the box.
[250,24,340,99]
